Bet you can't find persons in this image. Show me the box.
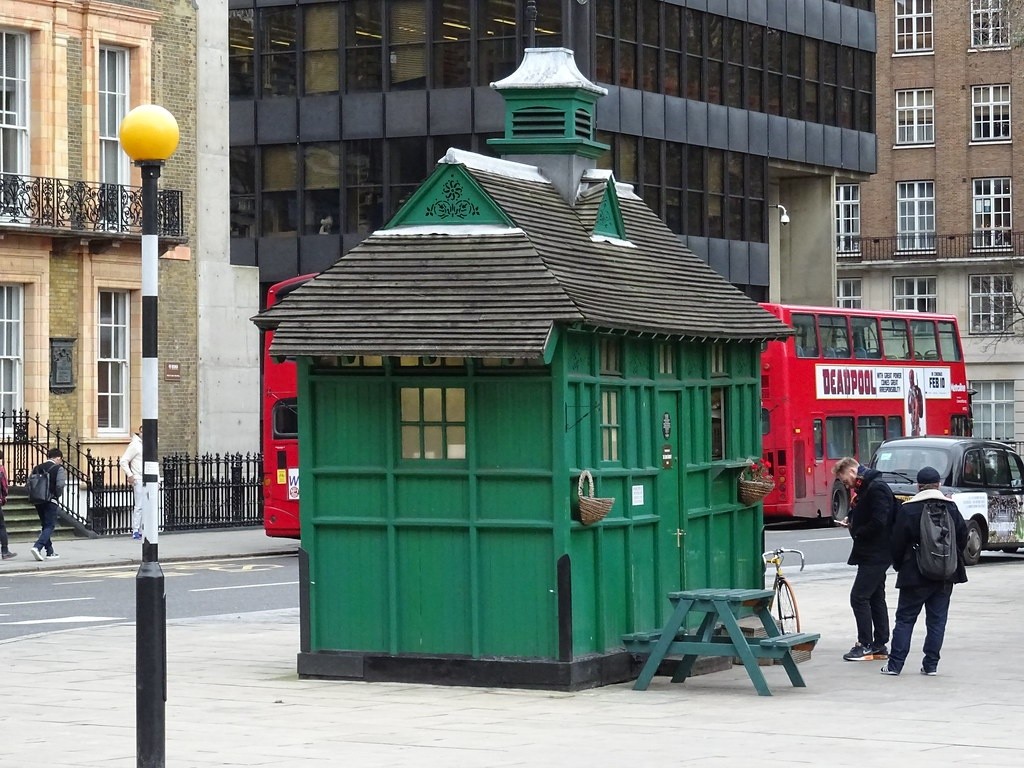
[25,448,64,559]
[0,450,17,560]
[119,426,143,540]
[908,369,923,436]
[831,457,896,661]
[880,466,968,675]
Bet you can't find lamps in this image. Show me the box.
[778,205,790,226]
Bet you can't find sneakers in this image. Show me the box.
[880,665,897,675]
[921,667,936,676]
[843,642,874,661]
[873,642,888,659]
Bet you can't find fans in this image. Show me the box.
[320,216,334,234]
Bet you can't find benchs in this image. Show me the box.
[620,627,820,697]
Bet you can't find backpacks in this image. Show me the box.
[912,501,957,580]
[27,465,58,504]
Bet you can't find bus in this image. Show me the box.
[261,272,323,541]
[716,303,978,526]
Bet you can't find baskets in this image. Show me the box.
[740,468,775,506]
[578,470,615,526]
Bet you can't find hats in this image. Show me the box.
[917,467,940,484]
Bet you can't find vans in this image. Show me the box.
[850,435,1024,566]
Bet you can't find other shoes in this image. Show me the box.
[133,532,141,540]
[2,552,17,560]
[46,553,59,559]
[31,547,44,561]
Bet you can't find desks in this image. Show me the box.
[630,589,807,697]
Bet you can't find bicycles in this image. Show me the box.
[761,546,804,634]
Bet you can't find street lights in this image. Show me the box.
[118,103,181,768]
